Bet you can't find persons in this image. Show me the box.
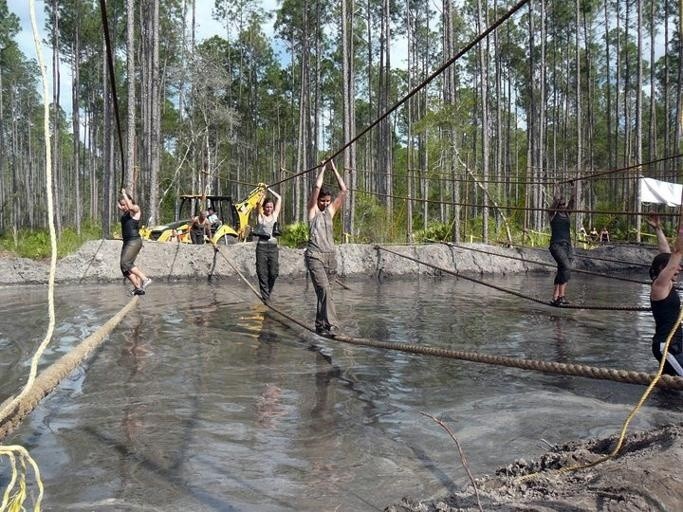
[589,227,598,242]
[114,187,153,298]
[255,310,291,426]
[599,226,609,246]
[166,227,181,242]
[303,154,346,337]
[206,207,218,233]
[189,210,210,245]
[642,210,683,381]
[546,177,576,305]
[253,184,282,306]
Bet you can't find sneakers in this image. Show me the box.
[557,296,570,304]
[327,326,340,336]
[316,327,329,337]
[141,278,152,288]
[550,298,561,307]
[130,287,145,295]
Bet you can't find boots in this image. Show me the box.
[273,222,282,236]
[252,223,270,240]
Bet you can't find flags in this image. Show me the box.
[640,175,682,209]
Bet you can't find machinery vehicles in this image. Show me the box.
[136,182,271,244]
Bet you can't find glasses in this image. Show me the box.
[559,202,565,206]
